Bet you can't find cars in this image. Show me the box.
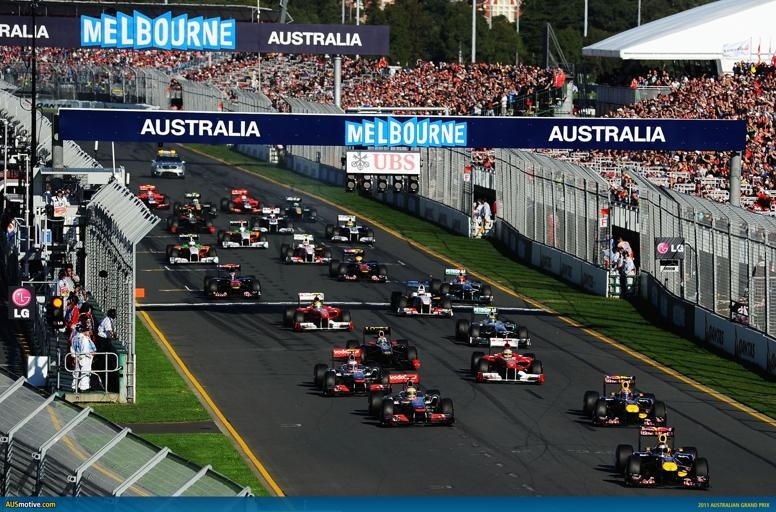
[150,149,186,180]
[615,424,711,489]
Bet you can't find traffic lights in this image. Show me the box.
[49,296,64,322]
[393,174,403,192]
[408,174,418,193]
[346,173,355,192]
[377,174,387,192]
[363,174,372,191]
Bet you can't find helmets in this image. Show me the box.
[621,388,632,400]
[656,445,668,458]
[503,349,513,358]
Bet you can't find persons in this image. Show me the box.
[487,312,498,321]
[463,198,638,304]
[620,389,631,401]
[313,300,322,309]
[657,444,669,457]
[0,42,775,220]
[502,349,513,358]
[0,221,117,392]
[415,285,426,296]
[377,337,389,346]
